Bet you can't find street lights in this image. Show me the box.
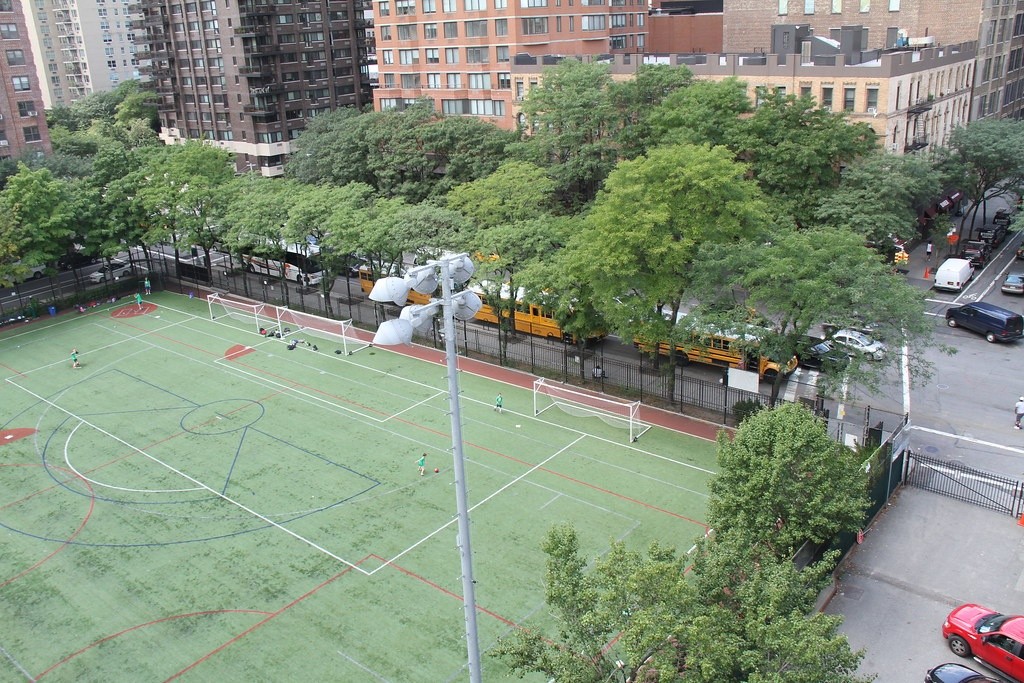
[368,252,482,683]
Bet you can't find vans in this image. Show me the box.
[945,302,1024,343]
[935,258,974,292]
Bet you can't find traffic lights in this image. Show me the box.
[894,251,909,264]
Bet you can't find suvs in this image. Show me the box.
[3,259,47,284]
[785,334,849,372]
[943,603,1024,683]
[963,209,1014,269]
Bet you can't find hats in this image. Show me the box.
[1019,397,1024,400]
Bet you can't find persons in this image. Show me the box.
[493,393,502,414]
[925,241,934,262]
[592,366,607,378]
[134,292,144,311]
[71,349,80,368]
[144,277,151,295]
[1014,397,1024,430]
[414,453,427,475]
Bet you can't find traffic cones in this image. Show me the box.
[924,267,929,278]
[1017,511,1024,526]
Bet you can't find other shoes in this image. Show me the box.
[1014,426,1020,429]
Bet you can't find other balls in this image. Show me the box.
[435,468,439,472]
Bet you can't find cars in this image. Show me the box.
[1017,245,1024,259]
[58,253,97,270]
[212,237,229,252]
[90,262,133,283]
[1001,272,1024,294]
[925,662,1004,683]
[307,251,367,278]
[822,314,888,361]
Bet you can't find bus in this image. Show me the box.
[238,234,332,286]
[633,310,800,384]
[359,266,608,345]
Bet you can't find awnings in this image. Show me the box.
[916,188,963,226]
